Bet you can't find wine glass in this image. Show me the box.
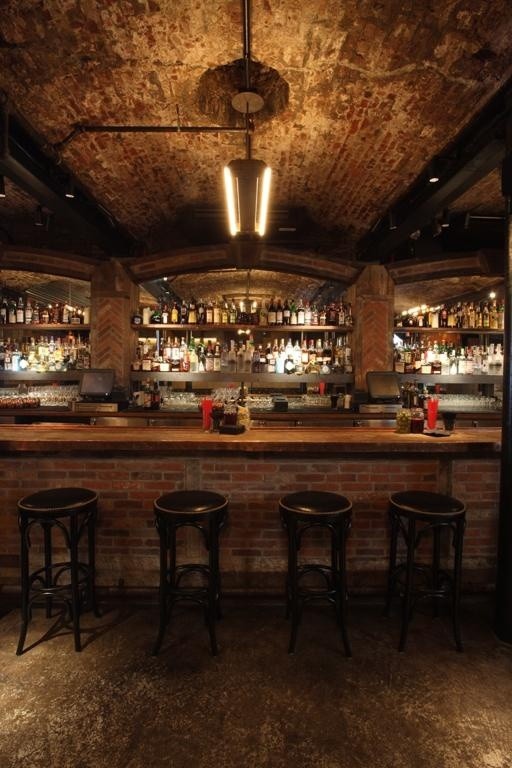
[209,387,241,408]
[0,385,81,407]
[424,393,500,413]
[248,393,331,410]
[161,385,201,414]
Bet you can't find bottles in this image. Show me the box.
[1,330,91,373]
[141,291,353,327]
[143,375,160,410]
[400,340,503,376]
[132,336,353,375]
[0,297,90,324]
[395,298,504,331]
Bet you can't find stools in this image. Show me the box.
[151,488,232,657]
[385,487,467,655]
[275,491,357,660]
[14,481,106,656]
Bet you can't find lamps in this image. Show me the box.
[220,90,274,243]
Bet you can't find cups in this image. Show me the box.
[329,391,352,412]
[410,417,424,433]
[441,411,456,432]
[425,397,438,431]
[297,380,326,396]
[197,397,224,433]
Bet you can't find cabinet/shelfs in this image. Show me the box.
[130,314,356,391]
[0,307,91,383]
[93,417,502,427]
[392,319,504,386]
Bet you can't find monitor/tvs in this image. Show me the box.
[366,371,401,404]
[79,368,115,402]
